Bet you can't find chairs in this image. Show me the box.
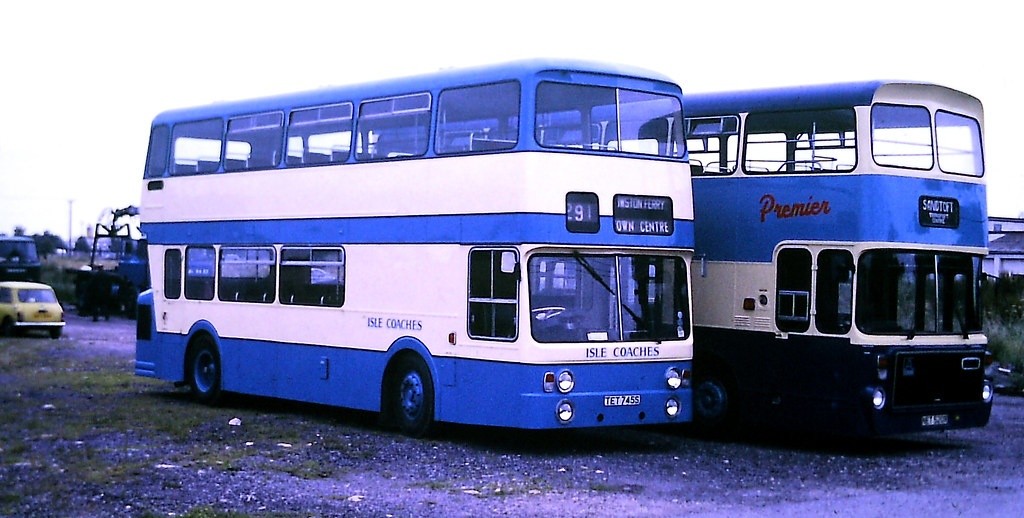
[690,158,705,176]
[176,281,344,307]
[172,127,490,175]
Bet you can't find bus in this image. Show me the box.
[130,57,706,440]
[366,79,1000,434]
[0,235,42,289]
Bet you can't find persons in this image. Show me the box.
[91,264,110,322]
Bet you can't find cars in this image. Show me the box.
[0,280,67,340]
